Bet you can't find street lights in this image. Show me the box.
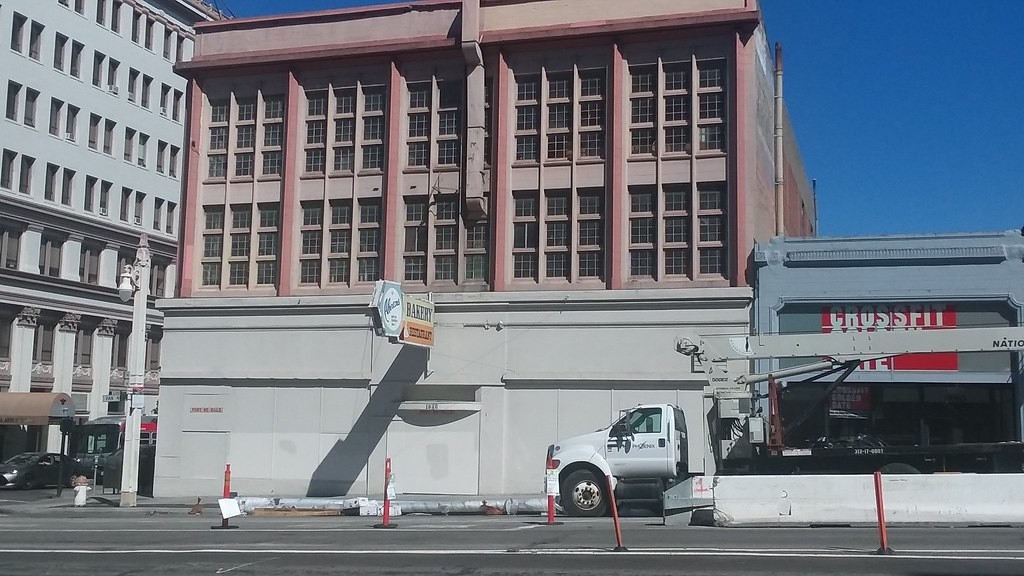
[116,264,146,508]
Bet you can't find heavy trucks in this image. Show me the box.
[543,328,1024,519]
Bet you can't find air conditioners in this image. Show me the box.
[128,92,135,101]
[160,107,167,115]
[135,218,142,225]
[66,133,76,141]
[99,207,108,215]
[108,85,119,94]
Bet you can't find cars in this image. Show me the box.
[0,452,68,488]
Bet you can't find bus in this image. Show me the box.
[66,415,157,484]
